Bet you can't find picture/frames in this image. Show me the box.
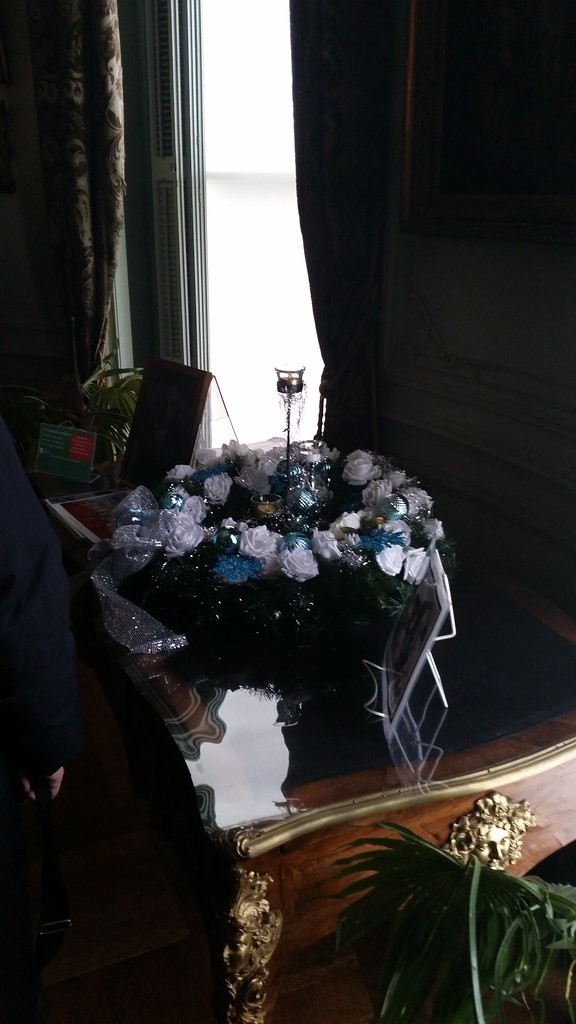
[381,545,453,744]
[115,355,215,490]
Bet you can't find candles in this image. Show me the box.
[257,499,277,517]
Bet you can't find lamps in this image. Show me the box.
[273,358,308,508]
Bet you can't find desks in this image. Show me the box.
[105,557,576,1024]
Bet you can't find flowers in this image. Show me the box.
[108,441,446,662]
